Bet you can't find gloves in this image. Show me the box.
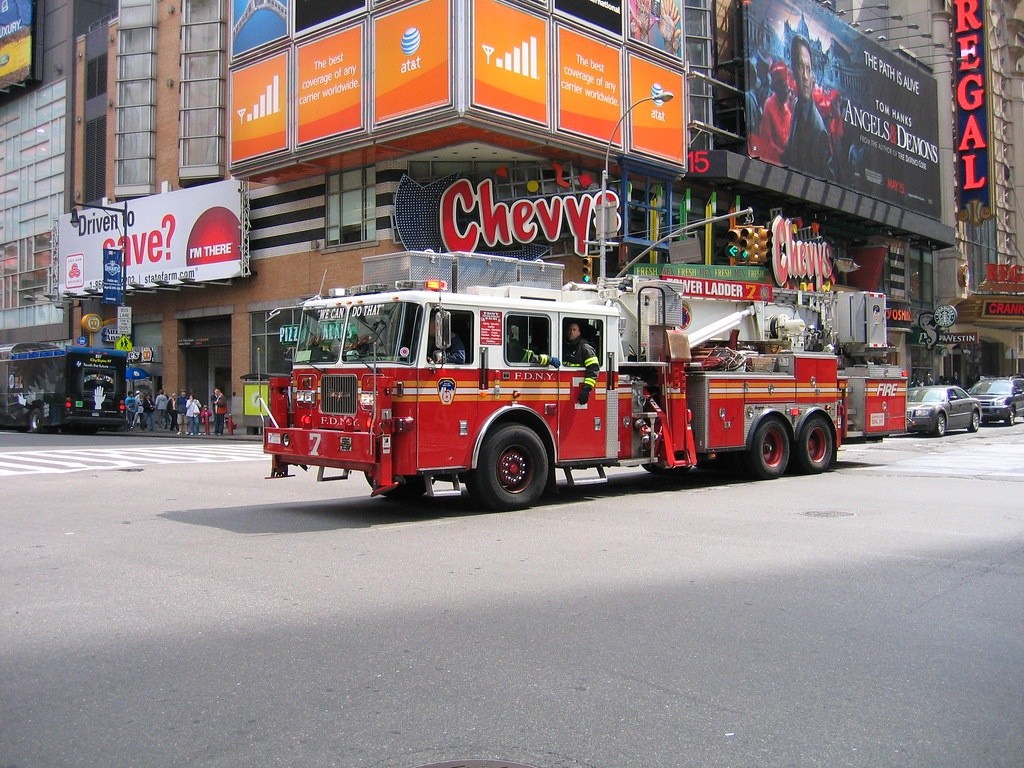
[549,357,560,368]
[578,386,592,405]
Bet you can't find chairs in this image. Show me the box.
[581,324,600,362]
[451,319,470,350]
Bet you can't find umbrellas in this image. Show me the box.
[126,367,152,397]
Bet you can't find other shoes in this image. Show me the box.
[197,433,201,435]
[185,432,189,435]
[191,433,193,435]
[176,432,182,435]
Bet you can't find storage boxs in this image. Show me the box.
[746,357,777,373]
[773,364,789,372]
[776,357,790,367]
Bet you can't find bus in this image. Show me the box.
[0,341,128,434]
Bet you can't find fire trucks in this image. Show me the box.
[255,247,917,511]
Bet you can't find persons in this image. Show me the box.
[131,390,142,430]
[125,391,138,430]
[912,369,919,382]
[426,313,465,364]
[924,373,934,386]
[211,386,228,436]
[164,388,189,435]
[744,34,843,182]
[201,405,213,435]
[155,390,168,429]
[507,323,560,369]
[141,394,155,431]
[186,393,202,436]
[562,322,600,405]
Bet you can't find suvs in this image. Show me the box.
[965,374,1024,427]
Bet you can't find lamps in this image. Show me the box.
[76,115,81,124]
[892,42,946,51]
[62,290,104,299]
[821,0,832,7]
[76,190,81,199]
[875,33,933,43]
[168,4,175,15]
[109,34,115,43]
[837,4,890,16]
[849,14,904,27]
[129,281,181,291]
[862,24,920,35]
[906,53,955,60]
[178,276,232,285]
[167,78,174,88]
[929,67,977,74]
[43,293,93,301]
[918,57,969,66]
[83,287,135,296]
[107,98,113,108]
[55,63,63,72]
[153,278,205,289]
[24,294,73,303]
[78,51,83,59]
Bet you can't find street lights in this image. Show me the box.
[68,199,129,350]
[598,90,676,277]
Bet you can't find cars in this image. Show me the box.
[906,384,984,438]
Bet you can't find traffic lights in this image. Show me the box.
[758,228,773,262]
[582,257,593,284]
[740,226,755,262]
[752,232,758,262]
[725,227,740,258]
